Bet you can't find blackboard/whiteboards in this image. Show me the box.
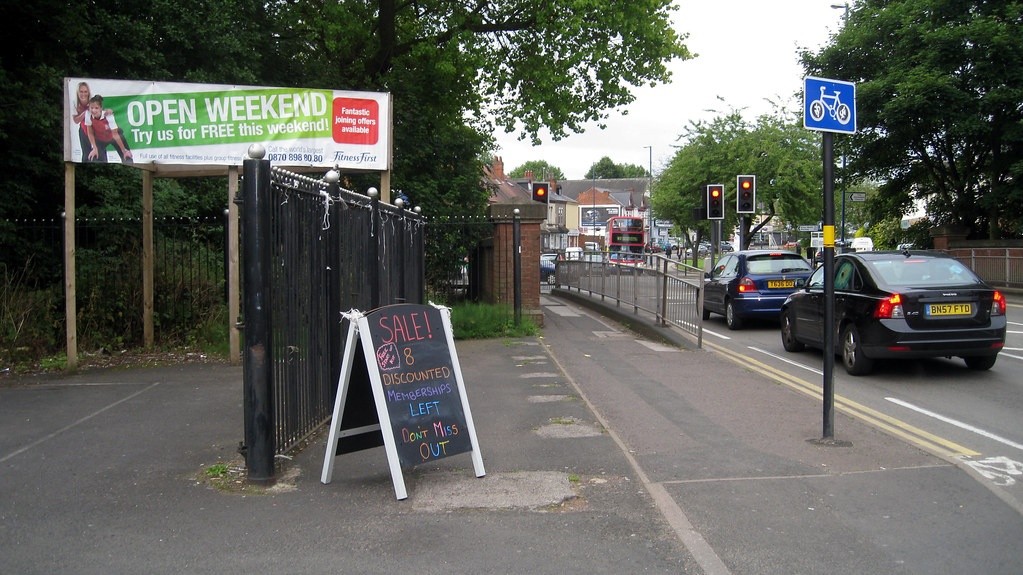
[324,301,478,464]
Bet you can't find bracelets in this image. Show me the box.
[122,148,128,154]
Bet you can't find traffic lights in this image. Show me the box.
[532,180,549,206]
[736,175,755,213]
[706,184,725,220]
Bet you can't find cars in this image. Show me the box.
[687,245,707,256]
[697,250,815,330]
[780,251,1007,375]
[541,254,565,270]
[540,257,568,285]
[585,242,601,254]
[705,240,733,252]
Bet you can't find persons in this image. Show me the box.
[645,242,653,265]
[71,82,133,167]
[677,247,682,260]
[666,246,672,259]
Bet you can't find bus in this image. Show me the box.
[604,217,646,273]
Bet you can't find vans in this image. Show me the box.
[645,242,660,253]
[565,247,584,261]
[844,237,874,253]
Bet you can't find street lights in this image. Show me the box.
[643,146,652,235]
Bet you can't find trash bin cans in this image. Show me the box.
[796,247,801,254]
[807,248,813,259]
